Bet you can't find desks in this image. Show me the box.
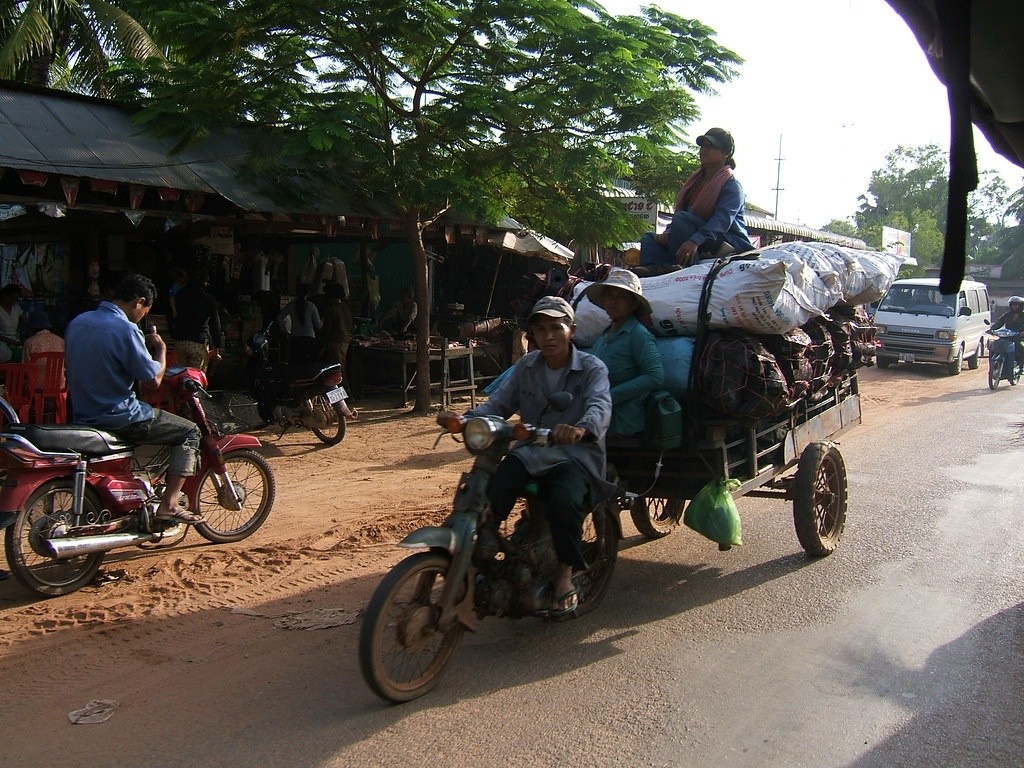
[355,341,503,408]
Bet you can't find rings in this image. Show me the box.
[686,253,691,256]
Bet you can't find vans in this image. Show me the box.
[872,278,991,376]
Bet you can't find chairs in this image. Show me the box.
[0,363,37,430]
[144,346,214,415]
[29,351,70,426]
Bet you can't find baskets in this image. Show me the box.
[987,338,1008,353]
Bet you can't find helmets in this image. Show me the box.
[1008,296,1024,313]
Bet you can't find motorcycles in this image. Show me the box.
[0,366,276,597]
[241,317,358,447]
[358,370,862,707]
[983,319,1024,391]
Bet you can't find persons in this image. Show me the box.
[277,283,358,403]
[436,295,613,617]
[986,296,1024,374]
[585,267,665,437]
[0,272,207,524]
[627,128,756,277]
[379,285,419,334]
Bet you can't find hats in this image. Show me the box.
[586,267,654,317]
[696,127,735,157]
[527,296,575,323]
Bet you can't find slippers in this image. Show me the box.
[551,582,582,617]
[156,510,205,524]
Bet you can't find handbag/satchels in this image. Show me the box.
[683,477,741,546]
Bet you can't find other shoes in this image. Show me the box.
[633,264,685,278]
